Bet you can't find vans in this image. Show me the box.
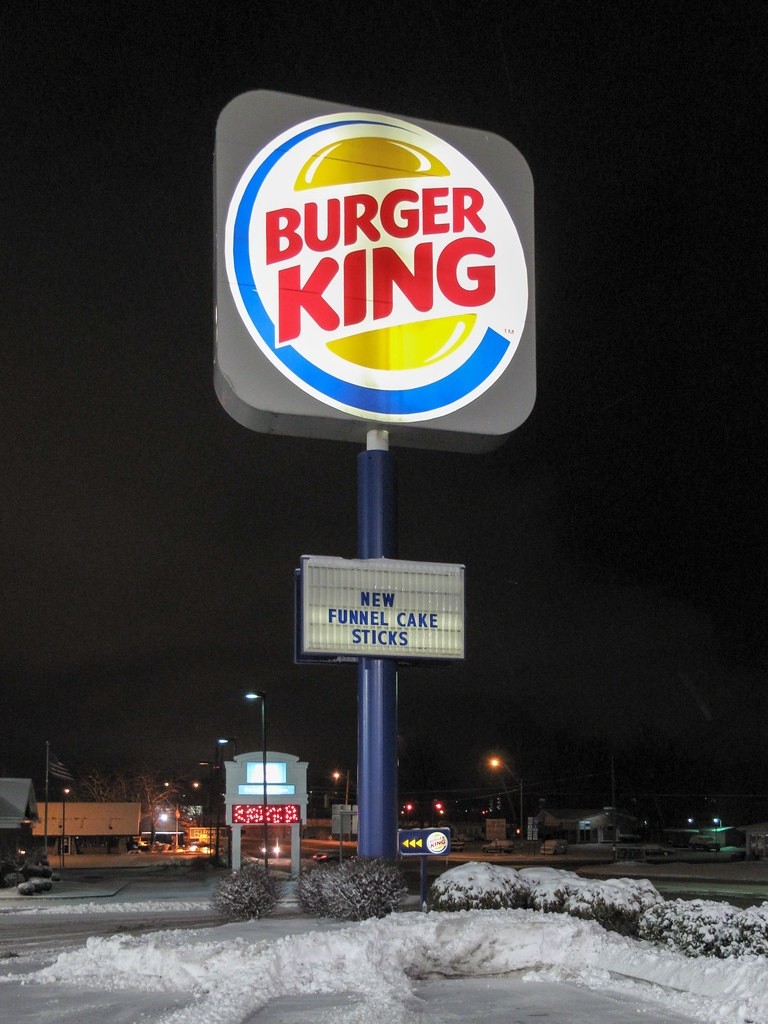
[541,840,569,855]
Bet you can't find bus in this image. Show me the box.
[142,832,184,850]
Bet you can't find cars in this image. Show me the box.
[313,849,349,865]
[659,838,688,848]
[450,838,464,852]
[257,841,283,858]
[646,848,674,856]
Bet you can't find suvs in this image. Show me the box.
[135,838,150,848]
[481,840,514,853]
[690,835,721,851]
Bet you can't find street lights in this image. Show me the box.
[193,784,212,861]
[714,818,722,828]
[165,782,178,848]
[246,691,269,879]
[491,758,522,843]
[63,787,70,868]
[334,769,348,803]
[199,761,220,858]
